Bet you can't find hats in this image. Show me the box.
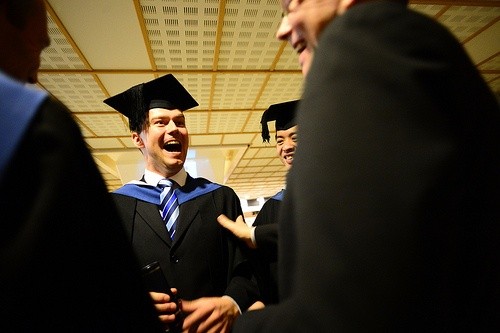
[102,74,199,133]
[260,98,304,144]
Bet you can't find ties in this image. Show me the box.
[159,179,180,240]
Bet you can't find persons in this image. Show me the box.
[104,73,255,333]
[217,0,500,333]
[0,0,164,333]
[244,100,302,311]
